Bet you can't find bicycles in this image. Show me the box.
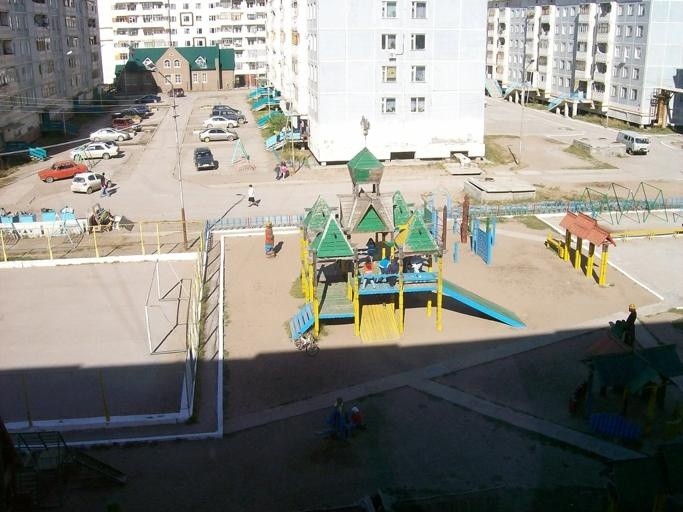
[294,329,320,357]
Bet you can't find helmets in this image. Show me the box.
[307,333,310,336]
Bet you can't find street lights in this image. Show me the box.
[360,117,369,147]
[59,50,72,142]
[86,27,113,110]
[517,12,535,163]
[142,59,188,252]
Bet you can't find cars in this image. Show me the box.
[134,94,161,104]
[194,147,215,170]
[36,160,88,183]
[111,105,151,132]
[70,140,119,161]
[89,127,130,142]
[71,171,112,194]
[199,128,238,142]
[201,115,238,128]
[170,88,184,97]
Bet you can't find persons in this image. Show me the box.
[410,255,422,275]
[275,161,288,182]
[361,256,376,290]
[378,255,391,284]
[350,407,367,431]
[325,396,349,444]
[365,238,378,262]
[97,172,110,197]
[622,303,637,346]
[246,185,258,207]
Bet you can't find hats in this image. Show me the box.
[368,256,373,263]
[629,304,636,308]
[266,222,272,227]
[352,407,359,412]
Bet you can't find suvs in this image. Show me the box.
[210,105,246,124]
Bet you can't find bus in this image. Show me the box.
[616,130,651,155]
